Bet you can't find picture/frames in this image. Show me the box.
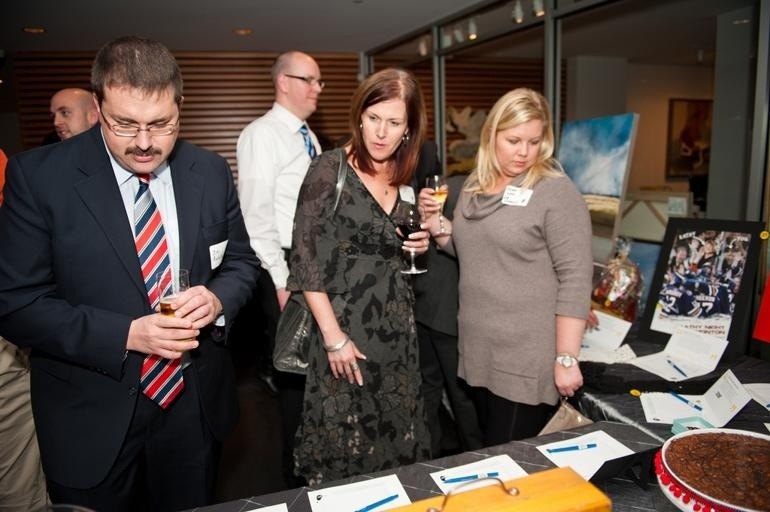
[665,98,712,181]
[637,217,763,363]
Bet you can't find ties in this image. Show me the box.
[134,172,185,408]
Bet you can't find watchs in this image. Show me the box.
[554,352,580,369]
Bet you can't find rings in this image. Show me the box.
[351,360,359,370]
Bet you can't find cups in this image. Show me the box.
[156,269,189,318]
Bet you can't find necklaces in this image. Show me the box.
[385,190,388,196]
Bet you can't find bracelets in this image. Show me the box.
[322,331,350,351]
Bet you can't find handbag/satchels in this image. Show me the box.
[272,296,313,375]
[537,395,594,436]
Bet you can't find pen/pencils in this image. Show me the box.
[667,359,688,378]
[444,472,499,483]
[547,444,596,452]
[356,495,398,512]
[670,390,702,411]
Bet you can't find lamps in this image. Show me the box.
[452,0,545,40]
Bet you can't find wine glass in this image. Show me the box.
[397,202,426,273]
[426,175,452,237]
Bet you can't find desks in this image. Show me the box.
[175,339,770,512]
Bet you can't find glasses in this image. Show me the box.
[285,74,324,88]
[100,108,180,137]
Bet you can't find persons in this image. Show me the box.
[418,86,594,447]
[234,50,326,492]
[285,66,431,487]
[49,87,100,142]
[659,226,752,318]
[0,33,260,512]
[0,148,54,512]
